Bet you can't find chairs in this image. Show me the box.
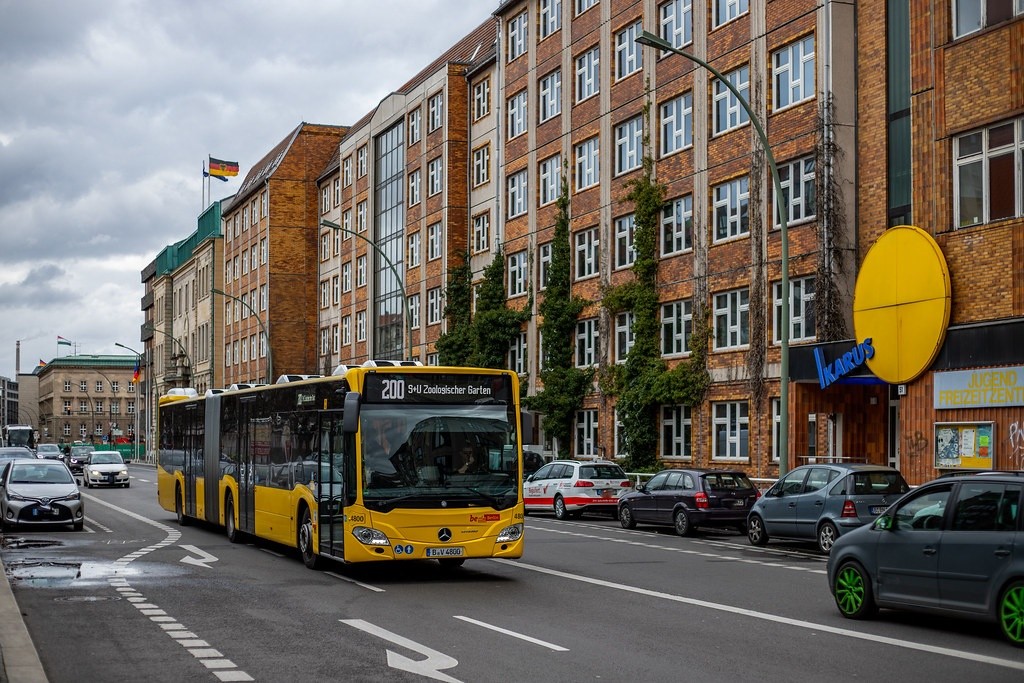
[15,470,26,480]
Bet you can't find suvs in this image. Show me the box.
[746,463,910,555]
[827,468,1024,648]
[488,450,546,483]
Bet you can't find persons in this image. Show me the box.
[63,444,71,454]
[449,443,485,474]
[366,420,417,487]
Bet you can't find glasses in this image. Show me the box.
[463,451,473,454]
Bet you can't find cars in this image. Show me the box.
[270,462,343,514]
[66,446,96,471]
[82,451,131,490]
[523,460,632,520]
[0,446,84,531]
[617,468,762,538]
[35,444,64,462]
[160,449,195,475]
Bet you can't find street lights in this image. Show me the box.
[145,326,195,388]
[14,382,94,443]
[94,370,117,433]
[320,219,412,362]
[633,31,792,478]
[114,343,158,456]
[209,289,273,383]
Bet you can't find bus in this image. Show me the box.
[2,423,34,450]
[156,360,525,570]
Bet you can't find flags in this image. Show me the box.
[58,336,72,346]
[133,367,141,382]
[40,360,46,367]
[203,162,228,183]
[210,156,239,178]
